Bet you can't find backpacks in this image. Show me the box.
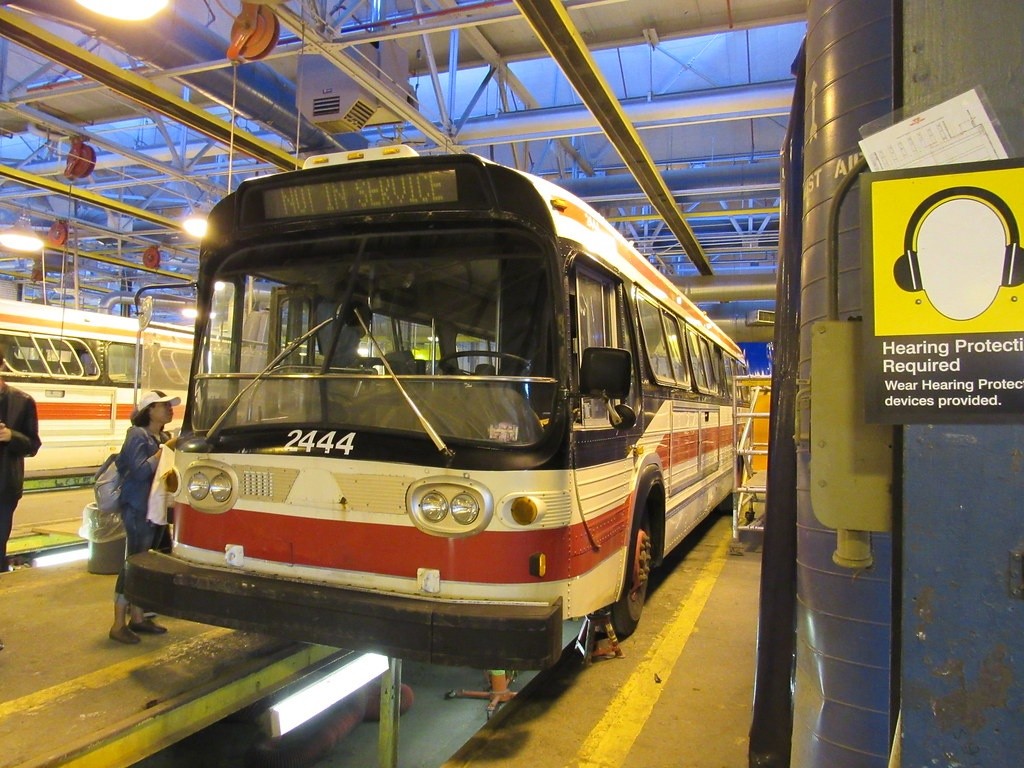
[92,426,150,514]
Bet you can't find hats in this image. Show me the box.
[138,390,181,411]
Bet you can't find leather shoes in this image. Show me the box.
[128,621,167,634]
[109,627,140,645]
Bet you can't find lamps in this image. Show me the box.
[184,198,218,240]
[0,208,46,253]
[181,302,199,319]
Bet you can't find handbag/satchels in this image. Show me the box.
[146,444,177,525]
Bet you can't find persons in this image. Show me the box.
[109,389,185,646]
[0,354,43,651]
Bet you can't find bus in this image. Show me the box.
[121,146,751,675]
[0,296,202,479]
